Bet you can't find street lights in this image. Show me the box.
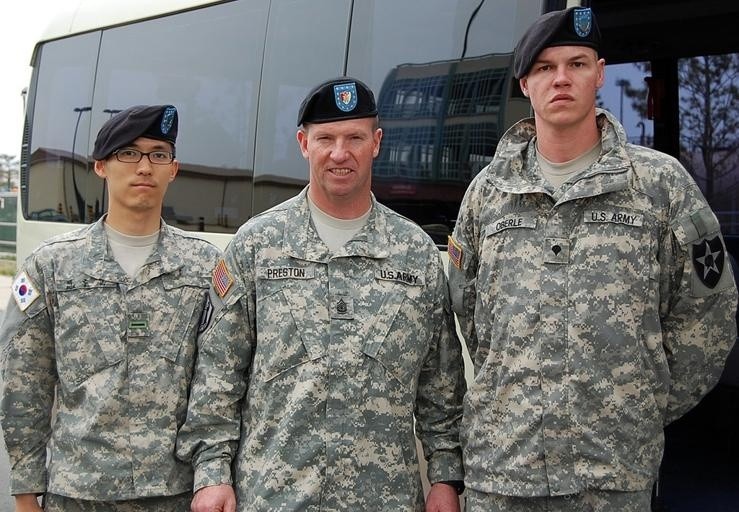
[614,76,630,125]
[70,105,90,222]
[101,107,122,219]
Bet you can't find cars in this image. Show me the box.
[29,208,69,222]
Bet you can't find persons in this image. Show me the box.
[0,104,223,511]
[444,5,738,512]
[171,77,465,512]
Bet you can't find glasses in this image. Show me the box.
[113,146,174,163]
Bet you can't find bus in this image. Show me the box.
[13,0,737,512]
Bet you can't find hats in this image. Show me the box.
[514,6,601,79]
[296,79,378,127]
[93,104,178,161]
